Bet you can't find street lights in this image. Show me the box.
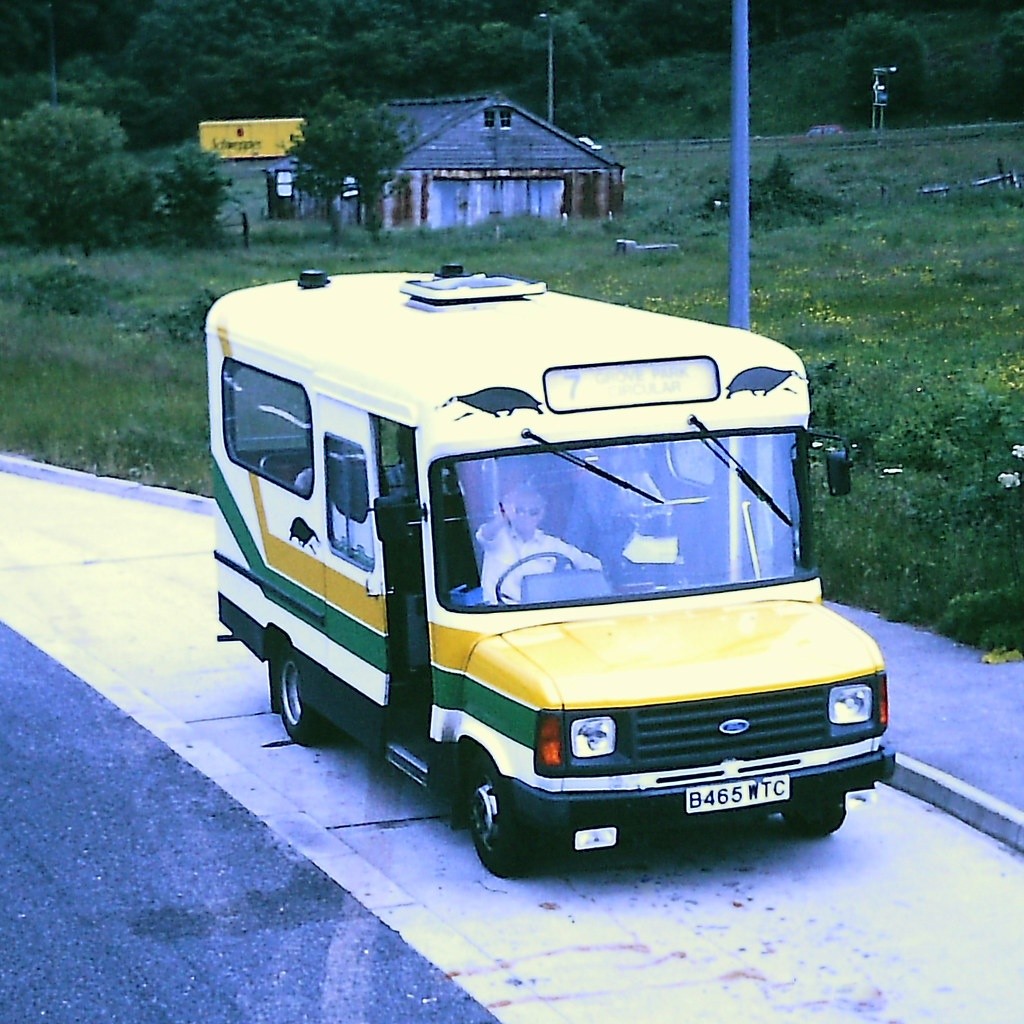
[537,14,558,125]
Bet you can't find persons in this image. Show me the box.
[474,485,602,604]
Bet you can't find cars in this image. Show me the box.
[793,124,846,149]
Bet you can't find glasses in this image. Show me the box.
[509,504,542,516]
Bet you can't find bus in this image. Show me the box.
[203,264,899,886]
